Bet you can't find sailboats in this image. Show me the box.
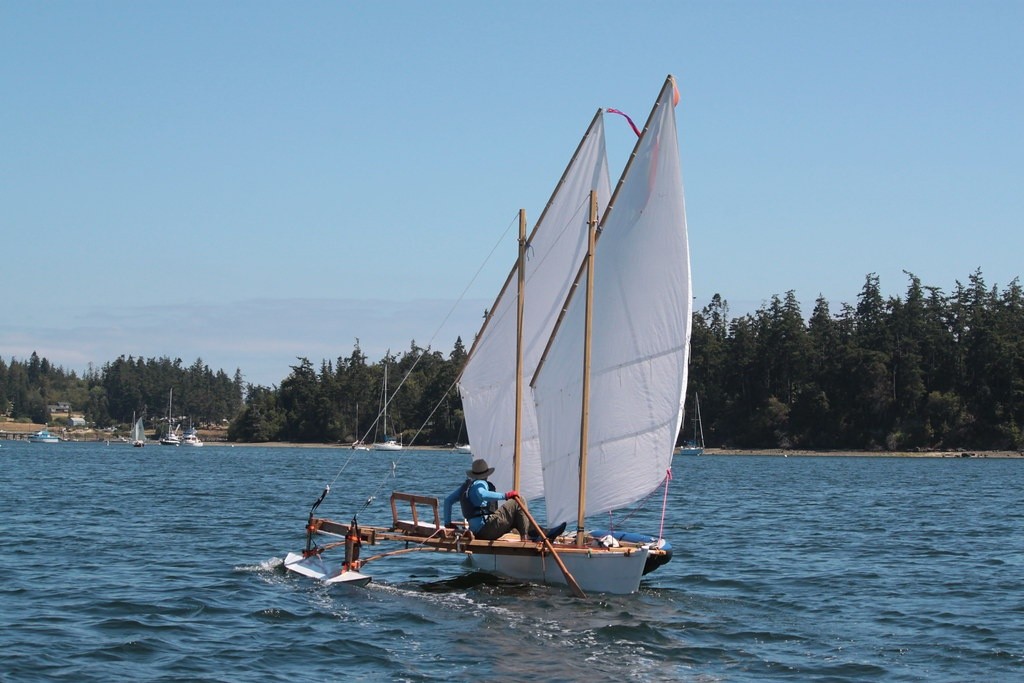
[352,401,367,450]
[282,75,693,595]
[372,365,402,451]
[680,391,704,456]
[454,415,471,454]
[130,411,145,447]
[159,387,180,446]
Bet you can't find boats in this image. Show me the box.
[28,422,59,442]
[181,417,204,447]
[102,435,128,443]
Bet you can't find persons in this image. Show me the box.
[444,458,567,545]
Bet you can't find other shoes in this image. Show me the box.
[545,521,566,544]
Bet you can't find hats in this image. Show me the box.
[466,459,495,480]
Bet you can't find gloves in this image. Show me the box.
[505,491,520,501]
[447,523,458,528]
